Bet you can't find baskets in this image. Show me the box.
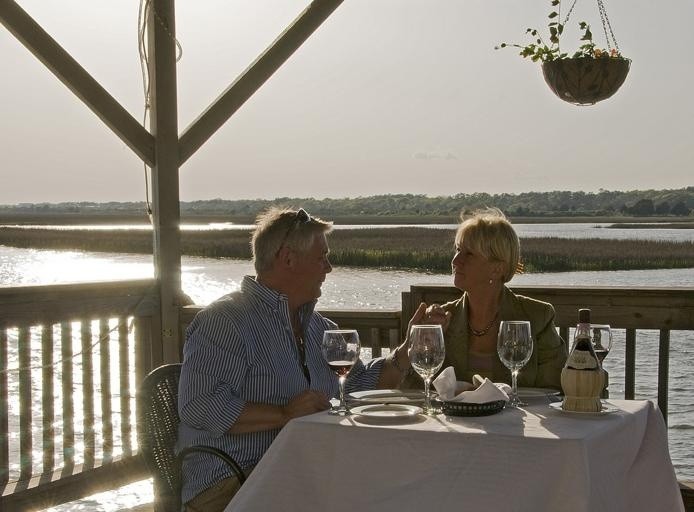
[443,400,505,417]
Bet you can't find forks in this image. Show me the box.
[545,390,561,402]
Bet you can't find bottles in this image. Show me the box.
[560,308,605,411]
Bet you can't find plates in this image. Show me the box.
[348,389,437,402]
[550,402,619,416]
[349,403,421,419]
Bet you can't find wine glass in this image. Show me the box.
[319,329,361,417]
[408,323,445,416]
[497,320,533,409]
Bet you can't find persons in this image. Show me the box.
[393,200,571,389]
[169,202,454,511]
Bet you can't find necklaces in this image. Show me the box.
[468,313,496,338]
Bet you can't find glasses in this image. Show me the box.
[277,208,311,256]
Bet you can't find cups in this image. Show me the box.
[574,323,613,363]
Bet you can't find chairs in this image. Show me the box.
[137,364,247,511]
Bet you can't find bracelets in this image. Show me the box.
[401,337,413,350]
[389,347,413,372]
